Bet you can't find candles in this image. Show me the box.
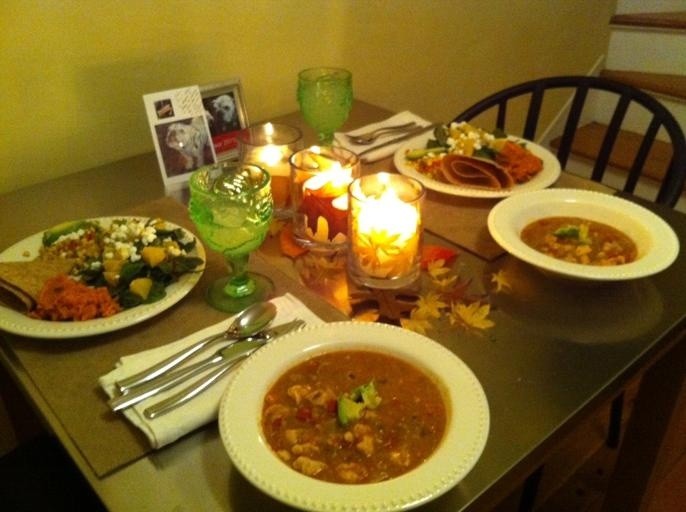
[241,122,423,287]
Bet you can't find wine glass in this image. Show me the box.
[298,67,350,144]
[187,162,275,316]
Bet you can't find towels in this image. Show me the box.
[330,109,432,164]
[93,291,326,454]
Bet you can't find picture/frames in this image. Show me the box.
[199,78,252,167]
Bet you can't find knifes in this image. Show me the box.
[106,322,305,415]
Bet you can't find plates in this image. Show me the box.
[221,321,491,511]
[1,215,206,341]
[487,189,682,284]
[395,128,563,197]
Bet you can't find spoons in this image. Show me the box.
[100,302,279,392]
[345,122,424,145]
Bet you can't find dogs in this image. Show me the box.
[211,93,239,133]
[165,116,211,171]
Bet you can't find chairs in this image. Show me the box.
[441,76,685,510]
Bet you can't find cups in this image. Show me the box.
[236,121,427,292]
